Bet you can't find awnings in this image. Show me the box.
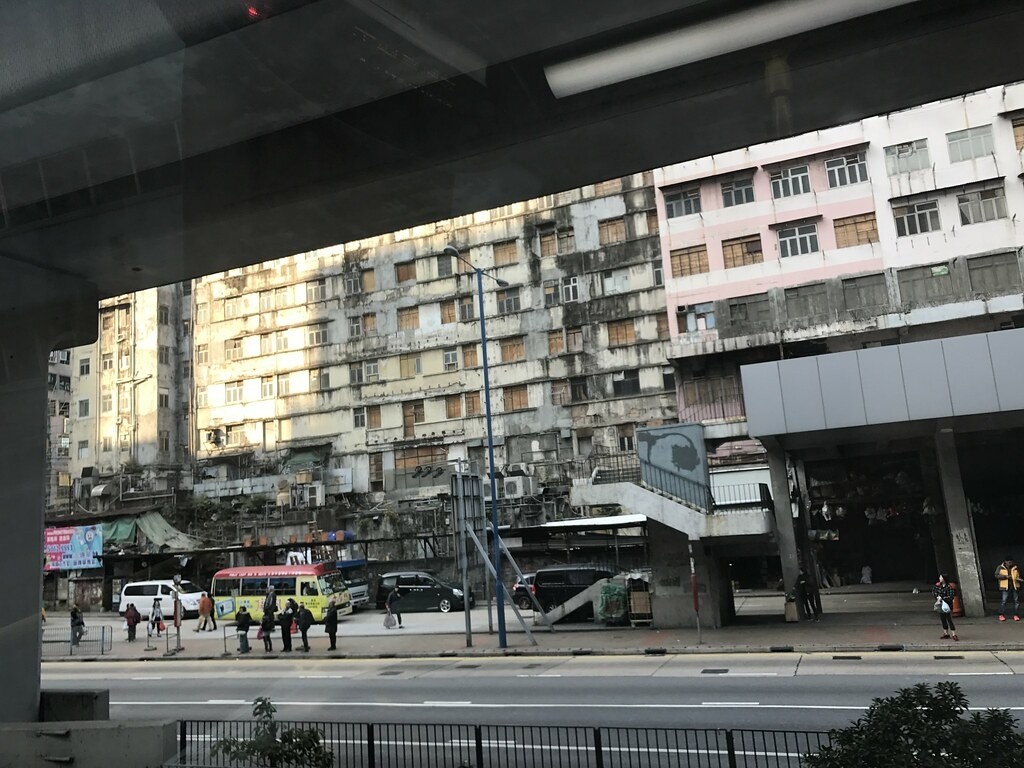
[93,534,453,584]
[91,484,111,497]
[195,451,254,476]
[277,433,341,459]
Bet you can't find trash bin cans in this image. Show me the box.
[949,582,963,617]
[290,618,298,634]
[785,592,800,622]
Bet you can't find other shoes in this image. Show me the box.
[953,635,958,641]
[399,625,404,629]
[237,646,337,654]
[940,635,950,639]
[158,634,161,637]
[807,618,820,623]
[386,626,391,629]
[194,628,216,632]
[271,629,275,633]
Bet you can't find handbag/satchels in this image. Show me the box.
[384,610,396,627]
[275,606,279,613]
[160,621,165,631]
[257,625,263,640]
[291,620,297,633]
[148,622,153,636]
[238,630,246,635]
[82,625,88,635]
[123,620,129,631]
[934,597,950,613]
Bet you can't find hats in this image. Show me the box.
[329,598,336,604]
[394,585,399,588]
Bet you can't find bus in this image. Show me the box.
[210,560,353,624]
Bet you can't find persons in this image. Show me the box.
[70,604,85,648]
[235,606,252,654]
[149,600,163,637]
[994,556,1023,621]
[125,603,139,642]
[793,567,820,623]
[260,585,338,654]
[193,593,217,632]
[385,585,404,629]
[932,574,959,641]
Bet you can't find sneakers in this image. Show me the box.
[1013,615,1020,621]
[998,615,1005,620]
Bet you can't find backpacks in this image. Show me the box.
[131,610,141,624]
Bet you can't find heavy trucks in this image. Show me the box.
[316,559,370,613]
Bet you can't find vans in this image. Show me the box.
[531,564,647,622]
[375,572,473,613]
[119,580,207,621]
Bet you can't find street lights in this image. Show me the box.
[442,245,510,649]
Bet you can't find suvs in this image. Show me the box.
[511,573,535,610]
